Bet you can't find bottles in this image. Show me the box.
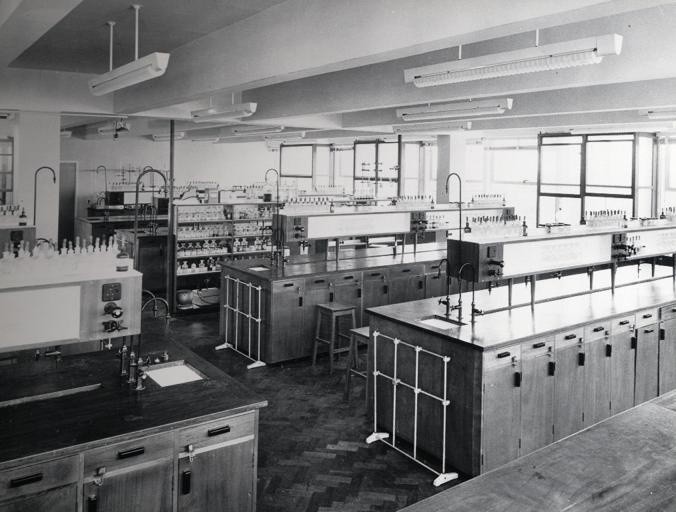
[580,212,586,225]
[627,236,637,256]
[177,208,273,275]
[116,236,130,272]
[330,198,336,213]
[623,209,628,228]
[659,208,666,219]
[431,196,435,209]
[19,207,28,226]
[464,216,471,233]
[522,216,528,236]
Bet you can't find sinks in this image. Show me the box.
[85,218,101,221]
[139,359,209,388]
[129,230,146,234]
[247,264,271,272]
[419,314,466,330]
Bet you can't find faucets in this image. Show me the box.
[436,258,475,314]
[134,169,168,254]
[34,166,56,224]
[445,171,462,240]
[96,166,107,196]
[261,227,285,266]
[122,290,157,347]
[264,169,279,241]
[97,196,108,211]
[138,297,171,358]
[143,165,155,202]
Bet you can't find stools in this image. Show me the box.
[314,300,369,404]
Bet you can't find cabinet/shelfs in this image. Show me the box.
[171,202,274,314]
[270,264,446,364]
[0,411,259,512]
[478,303,675,474]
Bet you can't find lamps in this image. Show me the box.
[394,30,626,124]
[190,90,259,128]
[89,5,171,98]
